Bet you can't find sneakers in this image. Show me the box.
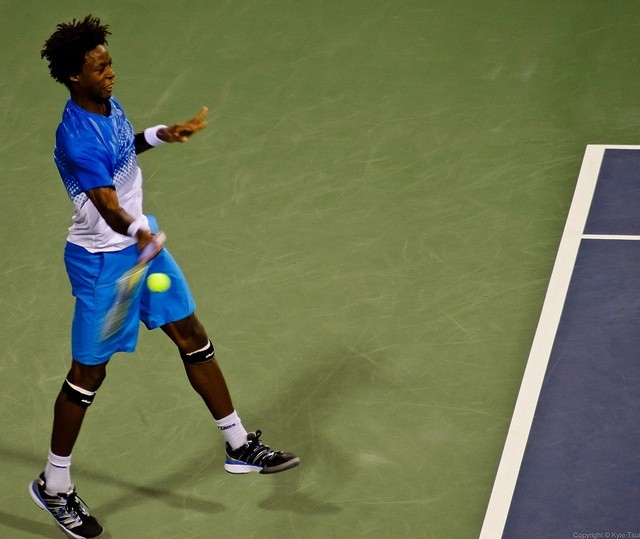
[29,471,105,539]
[224,429,301,475]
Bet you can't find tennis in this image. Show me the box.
[147,274,170,294]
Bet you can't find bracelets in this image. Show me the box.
[144,125,167,147]
[127,219,145,237]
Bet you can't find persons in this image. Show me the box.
[28,14,300,539]
